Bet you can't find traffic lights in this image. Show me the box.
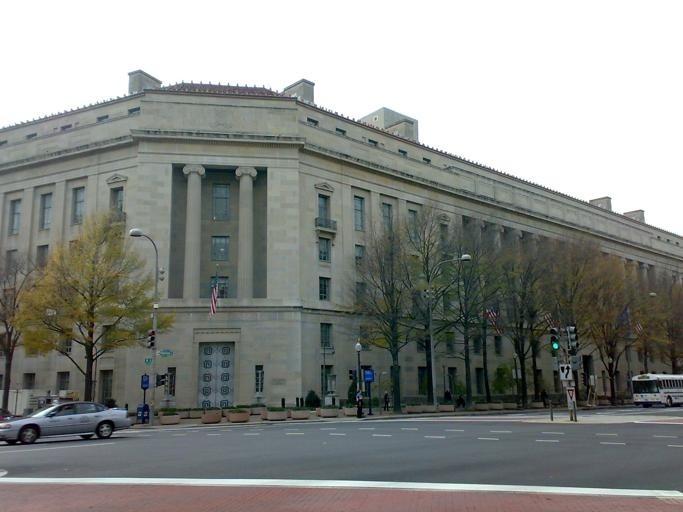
[565,324,579,351]
[146,329,156,349]
[156,374,167,387]
[549,324,561,357]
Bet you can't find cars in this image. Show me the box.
[0,387,130,446]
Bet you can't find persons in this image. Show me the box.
[355,389,366,418]
[541,388,549,409]
[383,389,389,411]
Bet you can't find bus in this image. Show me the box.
[631,372,683,409]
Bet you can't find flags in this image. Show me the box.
[207,271,218,322]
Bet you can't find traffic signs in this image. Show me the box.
[158,349,175,358]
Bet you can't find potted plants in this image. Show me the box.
[158,403,359,424]
[406,403,455,413]
[475,401,517,411]
[531,400,544,408]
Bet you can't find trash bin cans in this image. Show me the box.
[137,404,149,419]
[325,391,340,408]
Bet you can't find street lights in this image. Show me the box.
[128,227,168,425]
[608,356,617,406]
[610,289,658,366]
[512,352,522,410]
[427,253,472,411]
[354,338,366,418]
[378,370,387,415]
